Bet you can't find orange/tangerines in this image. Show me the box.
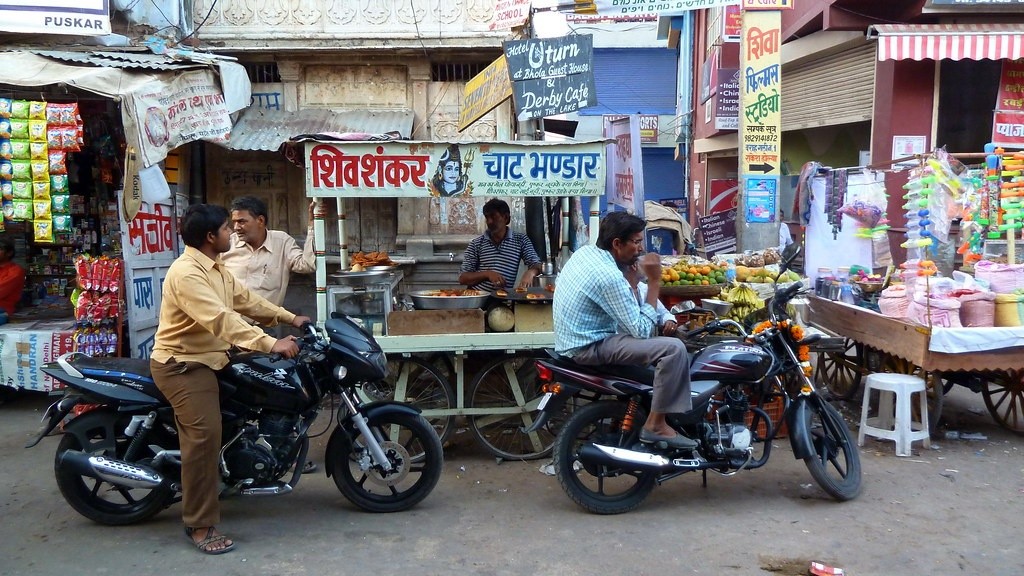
[660,263,727,286]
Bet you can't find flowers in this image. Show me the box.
[749,318,815,397]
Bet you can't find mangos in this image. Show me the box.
[736,265,801,282]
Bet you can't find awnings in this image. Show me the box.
[866,22,1024,63]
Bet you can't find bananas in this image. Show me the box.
[711,284,765,332]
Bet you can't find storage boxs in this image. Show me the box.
[726,388,787,440]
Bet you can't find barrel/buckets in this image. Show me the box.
[787,295,814,328]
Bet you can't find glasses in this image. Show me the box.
[627,238,643,246]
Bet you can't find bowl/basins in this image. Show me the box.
[327,265,395,285]
[359,298,384,314]
[701,299,734,317]
[409,290,490,310]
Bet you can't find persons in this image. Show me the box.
[150,203,312,554]
[552,211,698,449]
[458,198,542,293]
[779,209,794,256]
[0,239,26,327]
[217,196,328,475]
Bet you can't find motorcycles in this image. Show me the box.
[524,242,864,514]
[22,312,445,525]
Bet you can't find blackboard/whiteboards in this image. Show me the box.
[510,71,598,121]
[699,208,738,261]
[501,34,592,81]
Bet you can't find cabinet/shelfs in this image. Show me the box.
[326,270,408,335]
[23,176,123,294]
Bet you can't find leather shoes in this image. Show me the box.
[639,427,698,448]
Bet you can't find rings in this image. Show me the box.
[671,327,674,330]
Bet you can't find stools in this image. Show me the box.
[858,372,929,455]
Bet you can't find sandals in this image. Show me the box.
[185,526,235,555]
[288,457,317,474]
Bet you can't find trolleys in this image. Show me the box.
[291,134,620,461]
[802,295,1024,438]
